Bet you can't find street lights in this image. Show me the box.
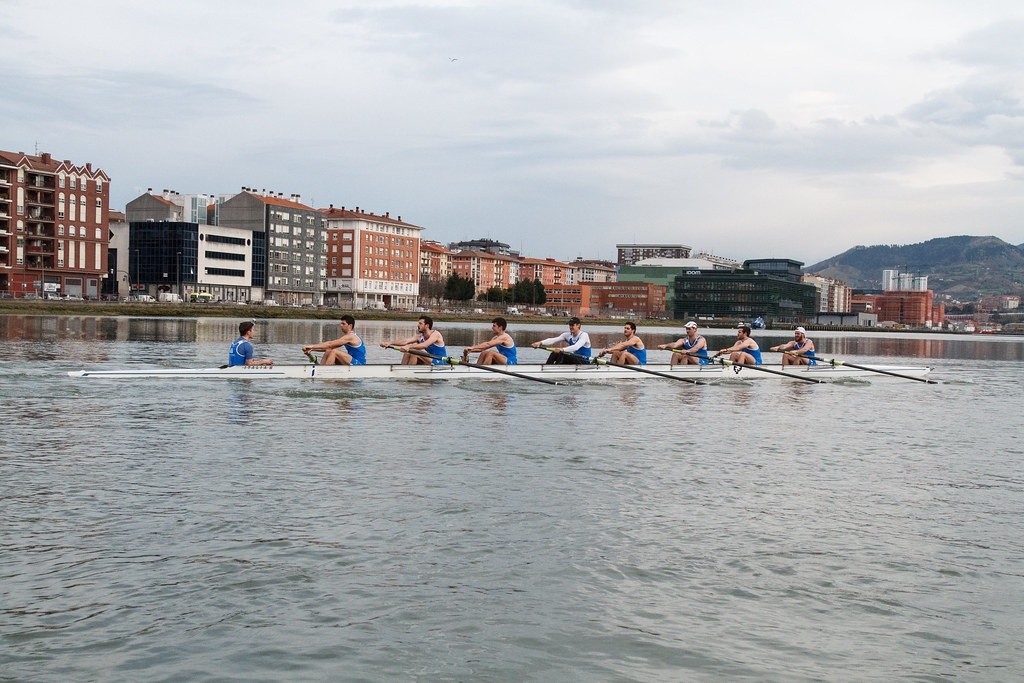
[134,249,139,302]
[176,252,182,294]
[108,251,115,294]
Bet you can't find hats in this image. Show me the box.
[683,321,697,329]
[566,317,580,324]
[795,327,806,337]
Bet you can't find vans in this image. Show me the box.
[159,292,184,303]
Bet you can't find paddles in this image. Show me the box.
[666,347,827,383]
[463,351,468,361]
[379,343,566,386]
[539,345,707,386]
[590,354,602,364]
[778,349,938,384]
[304,350,320,365]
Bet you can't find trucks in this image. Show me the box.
[134,294,158,303]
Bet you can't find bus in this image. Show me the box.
[190,292,214,304]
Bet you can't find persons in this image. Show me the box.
[229,322,273,366]
[532,317,592,365]
[381,316,447,365]
[770,327,816,365]
[719,327,762,365]
[464,317,518,365]
[302,315,367,365]
[600,322,647,365]
[658,321,708,365]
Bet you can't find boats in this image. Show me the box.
[63,360,936,385]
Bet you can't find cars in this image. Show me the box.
[65,295,86,302]
[24,293,44,300]
[44,292,64,301]
[0,293,15,300]
